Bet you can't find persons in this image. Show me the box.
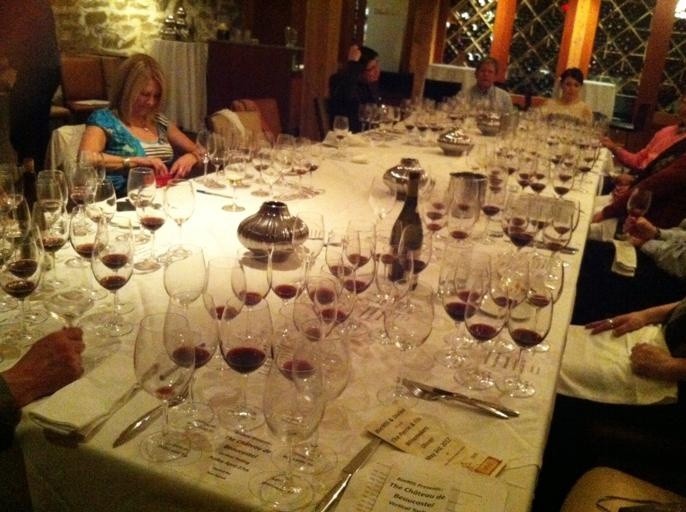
[571,215,686,327]
[0,7,78,221]
[0,324,90,453]
[587,82,686,242]
[80,50,210,197]
[327,42,405,136]
[543,287,686,412]
[544,66,594,127]
[454,54,514,118]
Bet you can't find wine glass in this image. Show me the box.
[614,187,653,244]
[0,89,608,512]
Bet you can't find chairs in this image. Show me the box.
[46,56,125,209]
[232,97,283,143]
[313,96,330,140]
[206,111,262,171]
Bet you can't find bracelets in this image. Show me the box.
[190,152,201,167]
[123,156,131,168]
[655,226,661,240]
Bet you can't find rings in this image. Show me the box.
[608,319,615,329]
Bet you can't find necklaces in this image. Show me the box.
[138,120,151,133]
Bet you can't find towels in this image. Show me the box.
[610,239,639,277]
[558,323,678,406]
